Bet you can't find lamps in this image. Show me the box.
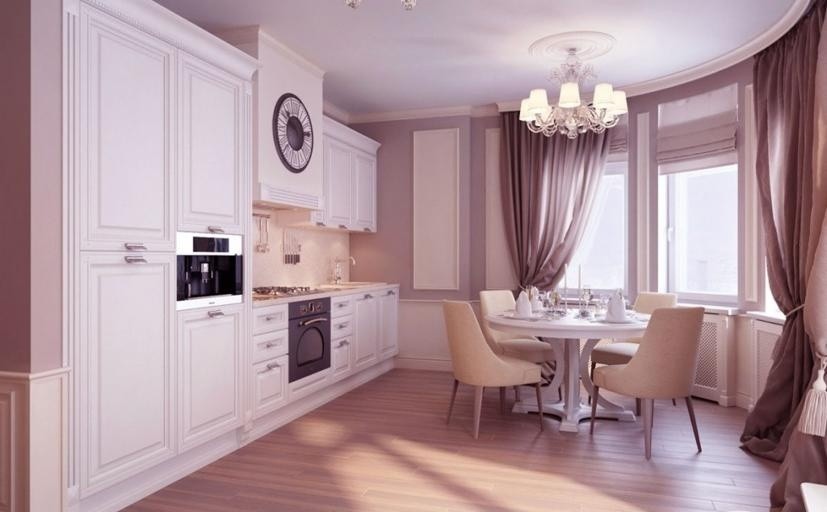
[519,49,627,139]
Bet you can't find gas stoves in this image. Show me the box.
[251,286,324,298]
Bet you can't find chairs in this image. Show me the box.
[442,299,545,439]
[480,290,562,402]
[590,307,705,458]
[589,292,676,416]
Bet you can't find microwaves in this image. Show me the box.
[175,231,244,311]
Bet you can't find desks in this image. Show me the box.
[484,309,652,433]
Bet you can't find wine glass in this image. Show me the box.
[525,284,629,320]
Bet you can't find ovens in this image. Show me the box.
[289,296,331,383]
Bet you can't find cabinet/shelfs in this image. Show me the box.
[175,303,246,438]
[253,285,354,403]
[176,51,246,234]
[72,3,174,250]
[279,114,382,233]
[87,266,166,483]
[354,288,399,362]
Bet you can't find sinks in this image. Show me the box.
[320,281,387,289]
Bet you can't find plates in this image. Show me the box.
[495,310,651,324]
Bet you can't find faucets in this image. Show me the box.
[334,256,355,285]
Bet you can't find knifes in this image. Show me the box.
[285,231,300,265]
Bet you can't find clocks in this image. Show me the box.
[272,93,315,173]
